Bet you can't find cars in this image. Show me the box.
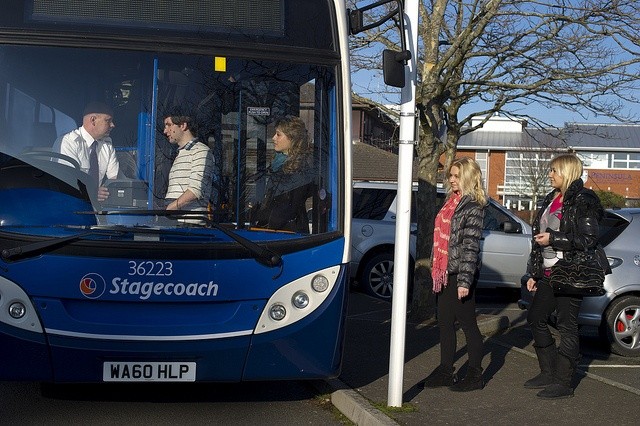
[518,207,640,357]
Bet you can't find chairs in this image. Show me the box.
[21,123,56,148]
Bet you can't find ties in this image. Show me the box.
[88,140,99,186]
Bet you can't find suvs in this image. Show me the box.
[351,179,532,302]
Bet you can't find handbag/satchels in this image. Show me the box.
[549,253,608,298]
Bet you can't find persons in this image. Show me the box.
[162,106,216,227]
[416,156,488,392]
[524,154,612,401]
[250,113,317,234]
[48,101,120,202]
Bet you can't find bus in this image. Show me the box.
[0,0,411,381]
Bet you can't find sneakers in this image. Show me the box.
[418,366,454,388]
[449,368,484,392]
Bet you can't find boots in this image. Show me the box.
[523,341,557,390]
[536,349,581,399]
